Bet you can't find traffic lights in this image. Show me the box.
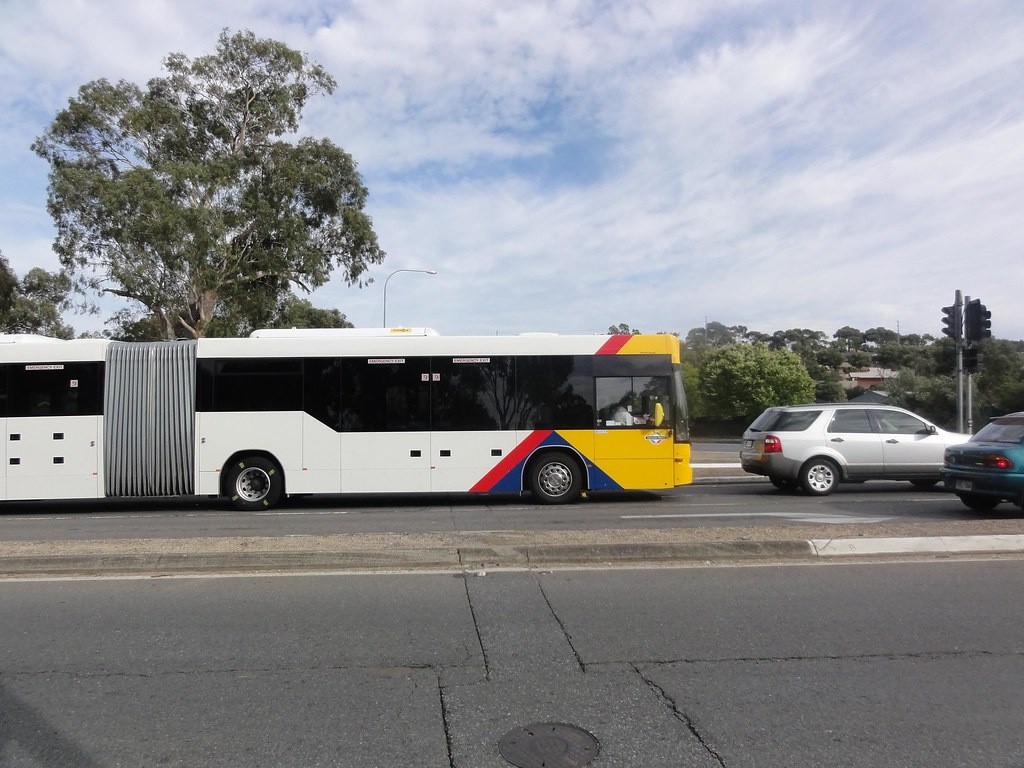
[941,306,956,339]
[963,347,984,375]
[967,299,991,341]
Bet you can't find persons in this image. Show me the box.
[609,394,648,426]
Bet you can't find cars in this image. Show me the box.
[940,411,1024,514]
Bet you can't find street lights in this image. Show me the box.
[383,269,438,328]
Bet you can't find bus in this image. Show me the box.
[0,325,693,511]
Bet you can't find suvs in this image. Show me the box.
[739,401,973,497]
